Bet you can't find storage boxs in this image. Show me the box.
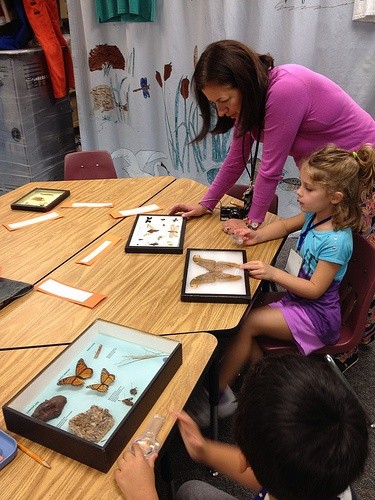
[125,213,185,253]
[11,188,71,213]
[2,317,183,474]
[180,247,253,305]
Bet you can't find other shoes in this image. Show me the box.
[331,345,361,374]
[183,382,238,428]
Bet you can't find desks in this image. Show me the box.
[0,176,290,500]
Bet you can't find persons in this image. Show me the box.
[166,40,375,375]
[114,352,370,500]
[181,145,375,428]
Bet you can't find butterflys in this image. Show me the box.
[138,215,181,246]
[57,358,93,387]
[189,254,243,288]
[85,368,116,393]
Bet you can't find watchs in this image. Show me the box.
[242,217,263,231]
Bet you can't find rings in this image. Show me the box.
[224,227,230,233]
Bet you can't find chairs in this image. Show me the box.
[225,184,279,292]
[227,231,375,438]
[64,150,118,181]
